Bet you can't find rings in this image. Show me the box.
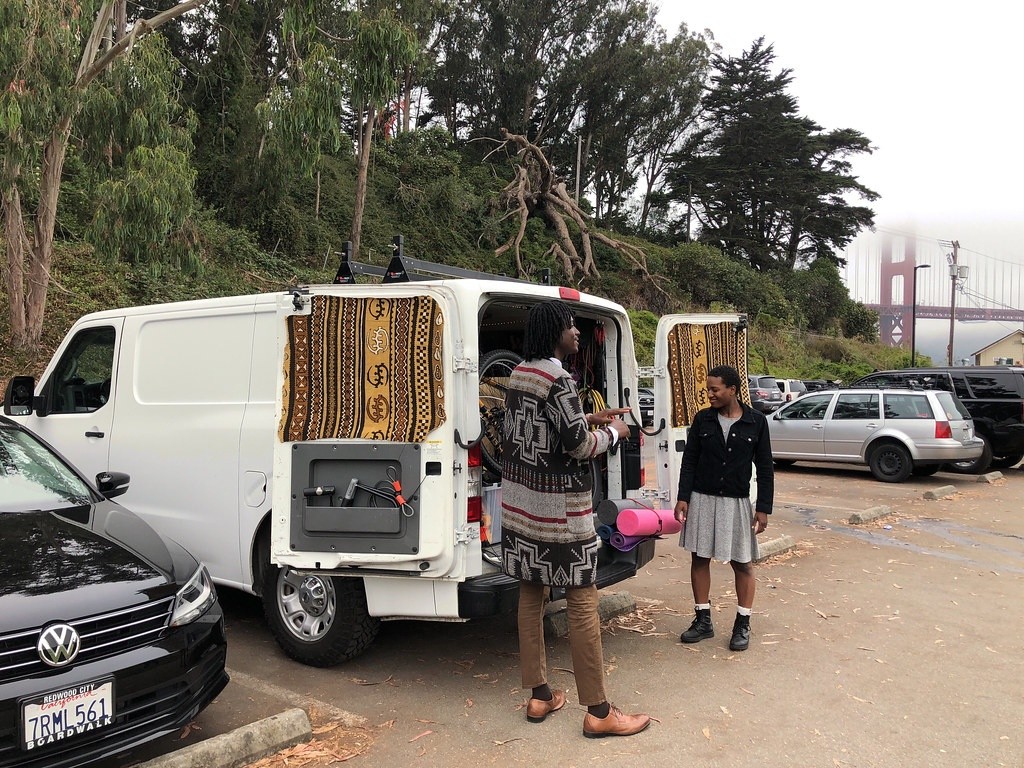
[764,528,766,530]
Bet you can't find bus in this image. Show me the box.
[804,379,829,393]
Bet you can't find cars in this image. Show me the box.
[747,375,783,414]
[638,389,655,429]
[0,415,231,768]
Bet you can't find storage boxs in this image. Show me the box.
[482,485,503,545]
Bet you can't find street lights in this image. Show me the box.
[911,263,931,366]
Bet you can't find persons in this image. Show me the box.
[674,365,774,650]
[500,299,651,738]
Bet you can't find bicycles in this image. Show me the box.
[478,349,602,514]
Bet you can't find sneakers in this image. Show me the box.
[681,605,714,643]
[729,611,751,650]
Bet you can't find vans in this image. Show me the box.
[0,235,751,668]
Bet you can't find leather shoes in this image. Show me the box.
[583,702,651,738]
[527,689,565,723]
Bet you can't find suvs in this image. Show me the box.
[841,364,1024,476]
[775,377,807,405]
[763,381,985,484]
